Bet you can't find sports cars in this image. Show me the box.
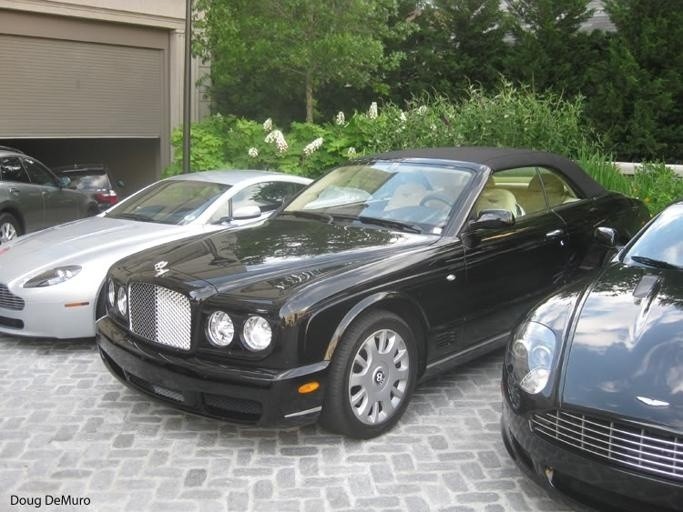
[1,169,317,341]
[502,199,682,509]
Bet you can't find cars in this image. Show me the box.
[59,163,125,213]
[1,147,101,242]
[94,147,654,440]
